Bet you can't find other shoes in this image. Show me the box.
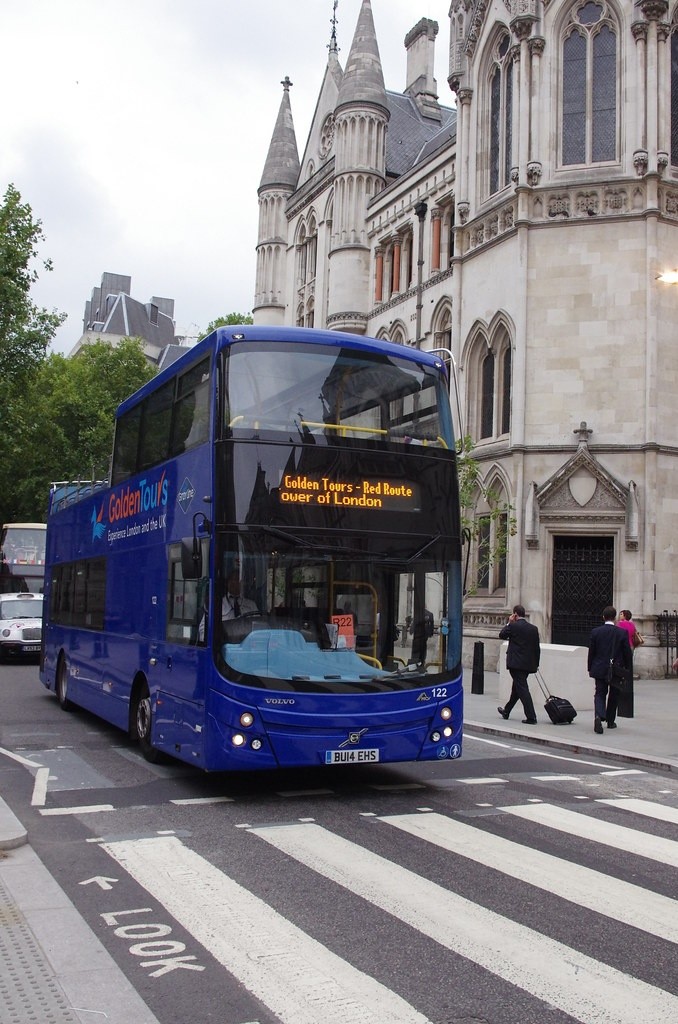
[607,720,617,729]
[498,707,509,719]
[594,716,603,734]
[522,718,537,724]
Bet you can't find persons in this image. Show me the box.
[198,569,260,642]
[344,600,359,635]
[616,610,637,653]
[411,603,434,664]
[587,607,632,733]
[497,605,541,724]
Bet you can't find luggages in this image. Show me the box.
[534,667,577,724]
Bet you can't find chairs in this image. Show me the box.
[58,478,109,511]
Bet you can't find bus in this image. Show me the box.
[0,522,48,595]
[38,325,465,777]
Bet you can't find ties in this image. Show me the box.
[233,597,241,617]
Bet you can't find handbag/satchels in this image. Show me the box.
[607,659,627,692]
[633,631,644,647]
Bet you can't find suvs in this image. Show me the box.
[0,592,44,663]
